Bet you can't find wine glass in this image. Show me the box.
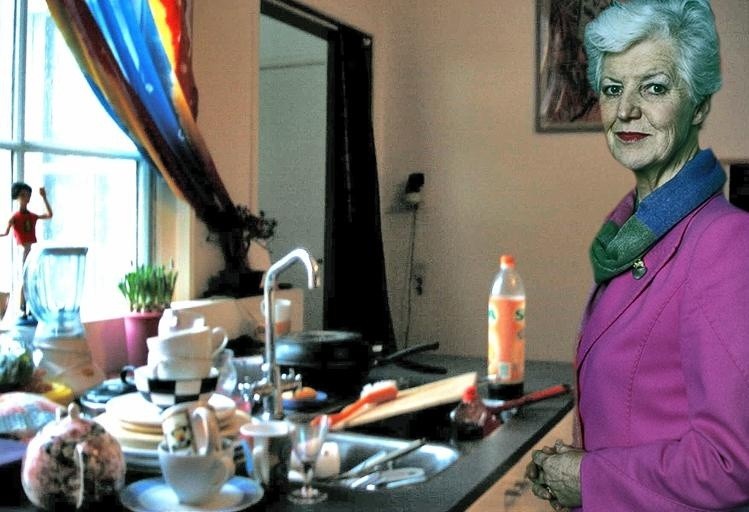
[283,415,332,503]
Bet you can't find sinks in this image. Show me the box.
[288,430,466,493]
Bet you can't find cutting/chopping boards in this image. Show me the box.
[329,371,477,430]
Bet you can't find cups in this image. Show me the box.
[238,422,294,496]
[156,440,235,504]
[121,308,237,413]
[261,299,293,338]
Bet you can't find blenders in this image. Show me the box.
[21,242,107,401]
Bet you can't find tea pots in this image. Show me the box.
[22,403,126,510]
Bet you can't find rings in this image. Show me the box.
[547,488,555,498]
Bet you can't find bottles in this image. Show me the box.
[487,254,526,399]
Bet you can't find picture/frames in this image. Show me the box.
[535,0,605,133]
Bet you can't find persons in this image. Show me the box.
[524,0,749,512]
[0,184,53,326]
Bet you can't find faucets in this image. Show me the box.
[245,245,319,420]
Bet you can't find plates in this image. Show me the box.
[120,474,265,512]
[91,392,260,473]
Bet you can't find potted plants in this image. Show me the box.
[117,257,180,368]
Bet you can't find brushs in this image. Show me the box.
[310,379,397,429]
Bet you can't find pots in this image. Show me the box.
[273,331,440,403]
